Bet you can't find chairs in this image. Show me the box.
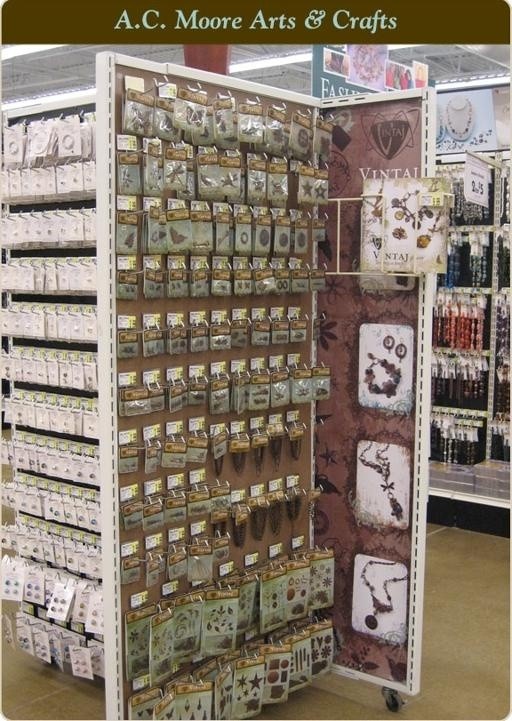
[0,51,510,720]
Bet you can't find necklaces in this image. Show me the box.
[358,441,405,521]
[445,97,473,136]
[365,350,402,399]
[360,560,408,630]
[432,171,510,469]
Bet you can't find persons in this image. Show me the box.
[386,64,425,90]
